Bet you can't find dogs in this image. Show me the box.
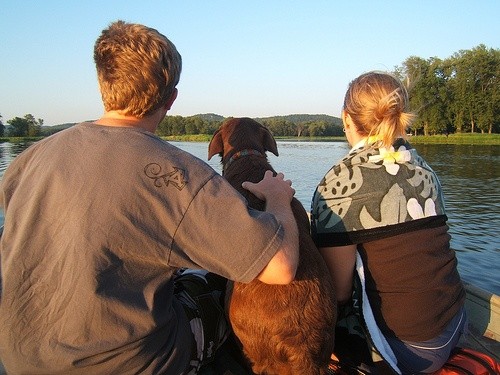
[207,117,337,375]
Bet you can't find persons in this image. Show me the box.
[0,20,299,374]
[310,70,467,375]
